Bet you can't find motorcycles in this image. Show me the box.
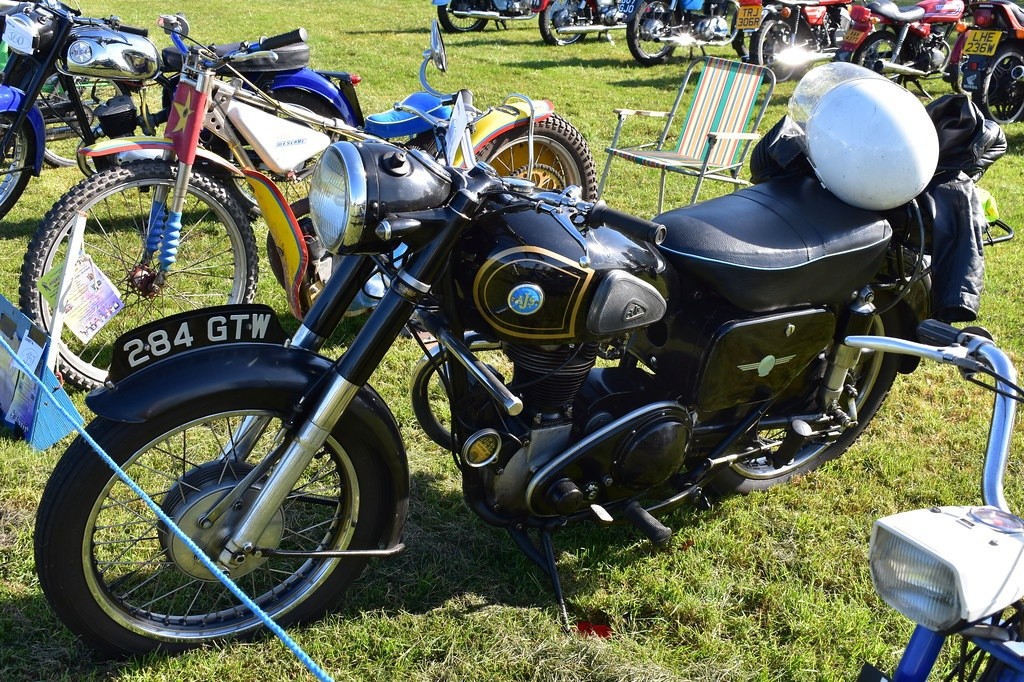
[0,1,1024,682]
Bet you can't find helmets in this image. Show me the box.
[805,76,940,210]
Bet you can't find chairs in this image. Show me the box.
[595,56,778,215]
